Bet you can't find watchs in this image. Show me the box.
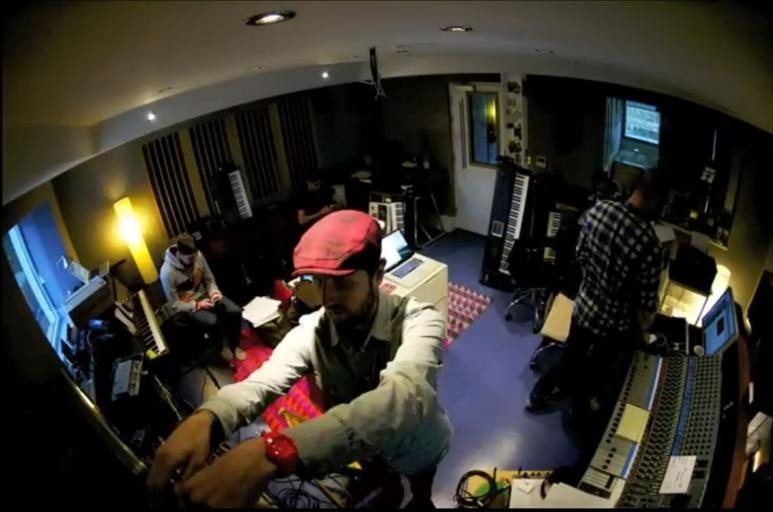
[259,429,299,482]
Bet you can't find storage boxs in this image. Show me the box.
[62,276,113,329]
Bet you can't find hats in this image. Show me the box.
[292,210,382,278]
[178,235,197,253]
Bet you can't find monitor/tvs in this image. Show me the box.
[693,286,738,363]
[621,99,660,147]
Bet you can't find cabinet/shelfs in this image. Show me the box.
[379,252,448,340]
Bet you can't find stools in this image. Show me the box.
[504,235,552,333]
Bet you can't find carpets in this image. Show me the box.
[439,280,494,349]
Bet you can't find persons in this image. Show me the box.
[564,165,674,387]
[161,234,246,361]
[146,210,453,511]
[298,170,345,225]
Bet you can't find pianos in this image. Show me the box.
[126,290,171,361]
[480,159,533,293]
[225,165,254,219]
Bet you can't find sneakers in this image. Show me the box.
[220,343,234,360]
[235,347,247,360]
